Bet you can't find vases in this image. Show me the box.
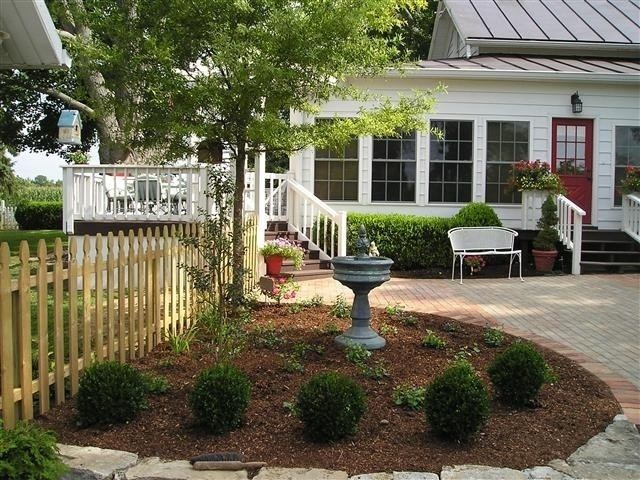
[263,253,284,275]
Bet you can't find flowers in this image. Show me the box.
[504,157,569,194]
[464,256,487,274]
[257,234,308,271]
[615,163,640,195]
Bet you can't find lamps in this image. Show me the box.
[567,87,585,115]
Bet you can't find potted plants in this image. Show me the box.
[531,194,565,274]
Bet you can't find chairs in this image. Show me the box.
[97,175,190,216]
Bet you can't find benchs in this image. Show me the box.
[446,223,527,283]
